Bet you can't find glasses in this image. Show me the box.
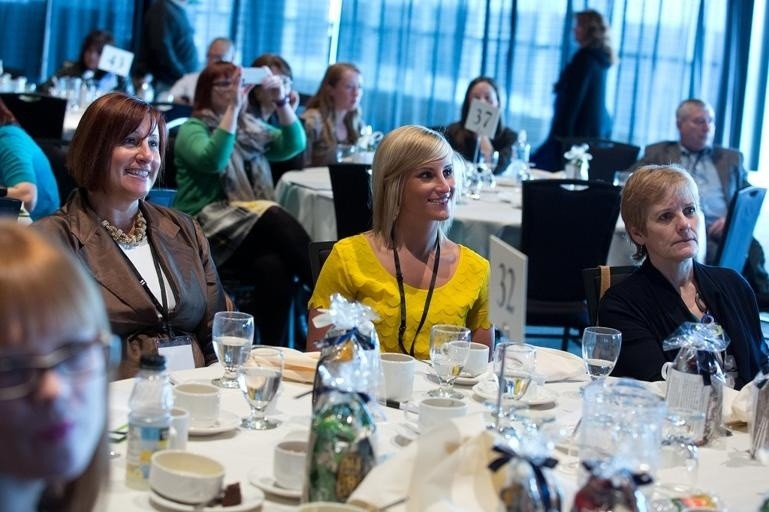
[0,336,125,404]
[209,80,234,87]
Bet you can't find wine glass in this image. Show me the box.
[128,310,768,512]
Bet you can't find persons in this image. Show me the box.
[306,126,492,362]
[0,217,112,512]
[444,77,517,174]
[546,10,612,170]
[645,100,769,312]
[598,163,769,391]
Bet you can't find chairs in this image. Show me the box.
[308,238,341,288]
[712,184,766,276]
[580,261,641,327]
[493,178,627,353]
[549,135,641,183]
[327,162,377,240]
[2,92,68,149]
[145,188,178,209]
[149,101,194,120]
[0,197,22,227]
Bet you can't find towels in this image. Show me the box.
[348,424,513,509]
[720,376,758,422]
[253,346,323,383]
[500,345,587,382]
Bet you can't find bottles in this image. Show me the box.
[0,61,173,114]
[334,129,630,205]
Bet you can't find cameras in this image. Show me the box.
[240,67,275,85]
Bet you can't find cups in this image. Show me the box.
[0,195,21,226]
